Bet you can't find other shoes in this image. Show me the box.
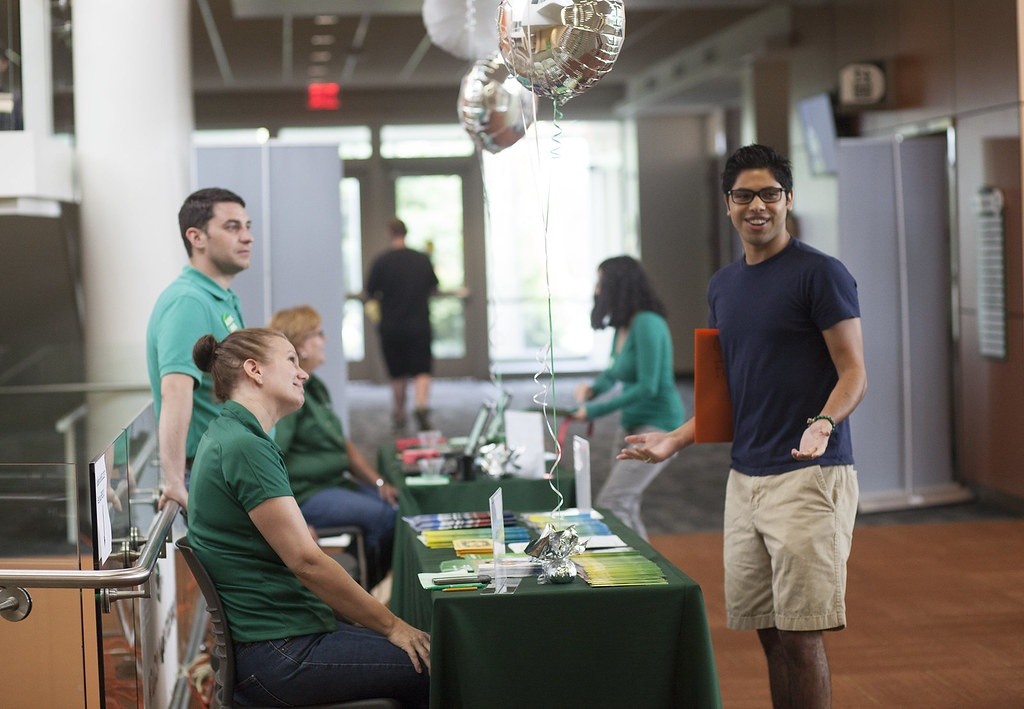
[392,412,407,428]
[416,408,431,430]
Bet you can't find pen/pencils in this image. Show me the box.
[427,583,487,590]
[442,587,477,591]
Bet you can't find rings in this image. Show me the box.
[644,457,651,463]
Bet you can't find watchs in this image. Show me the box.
[374,475,387,489]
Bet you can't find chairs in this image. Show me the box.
[174,536,401,709]
[315,526,369,593]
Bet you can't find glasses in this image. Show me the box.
[726,187,786,205]
[304,329,325,338]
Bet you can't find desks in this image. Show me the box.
[400,507,722,709]
[376,443,571,515]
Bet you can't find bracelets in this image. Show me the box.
[807,415,836,434]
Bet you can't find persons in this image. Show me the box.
[187,326,430,709]
[144,188,277,528]
[617,142,869,709]
[345,220,440,432]
[269,305,402,590]
[570,256,686,545]
